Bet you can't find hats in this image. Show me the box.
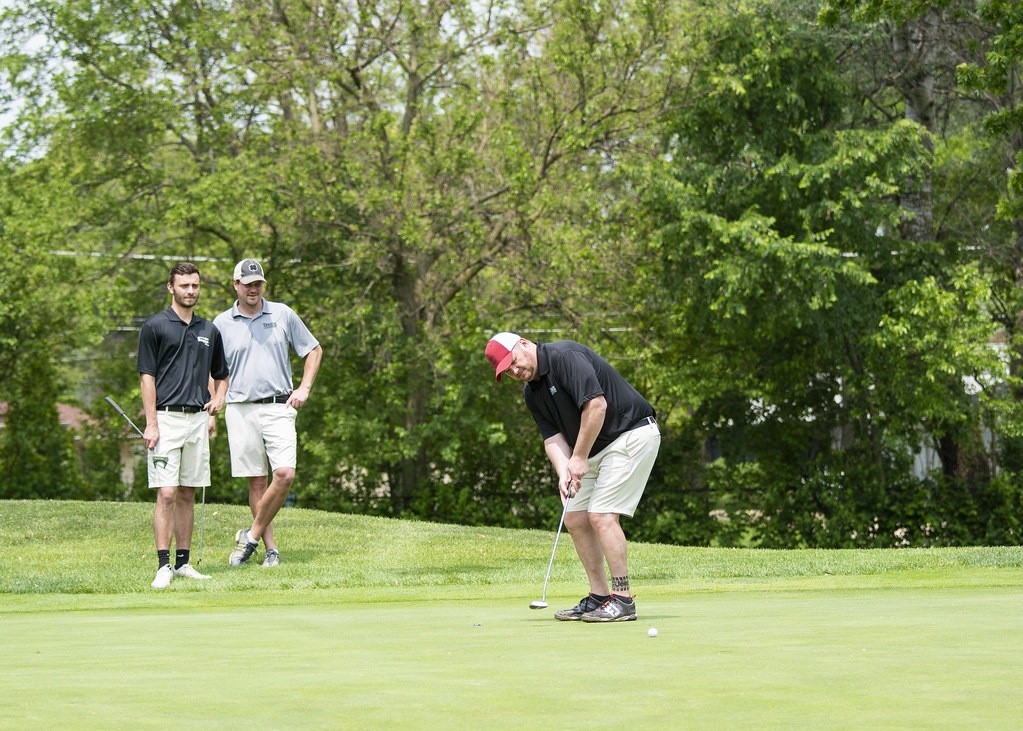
[234,259,267,284]
[485,332,521,382]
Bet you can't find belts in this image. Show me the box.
[157,405,205,414]
[252,395,289,403]
[626,416,655,429]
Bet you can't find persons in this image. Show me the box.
[485,332,661,623]
[209,259,323,569]
[136,262,229,590]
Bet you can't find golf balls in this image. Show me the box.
[647,627,659,637]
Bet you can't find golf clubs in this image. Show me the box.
[105,395,170,469]
[196,486,206,566]
[528,479,574,610]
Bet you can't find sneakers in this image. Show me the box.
[261,550,280,567]
[228,529,259,566]
[150,563,173,588]
[172,564,212,580]
[581,593,637,622]
[555,592,603,620]
